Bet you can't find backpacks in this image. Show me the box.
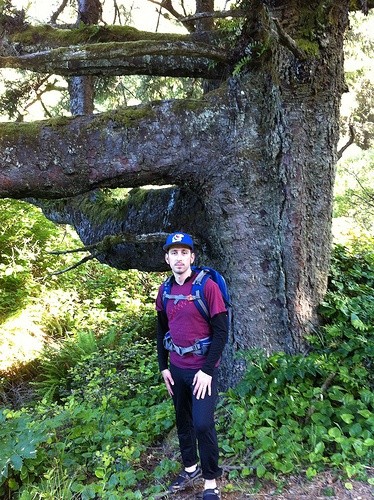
[162,265,229,320]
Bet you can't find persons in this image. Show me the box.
[155,232,230,500]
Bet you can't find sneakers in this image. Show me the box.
[171,464,200,488]
[202,488,219,499]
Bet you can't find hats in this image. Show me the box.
[163,232,193,254]
